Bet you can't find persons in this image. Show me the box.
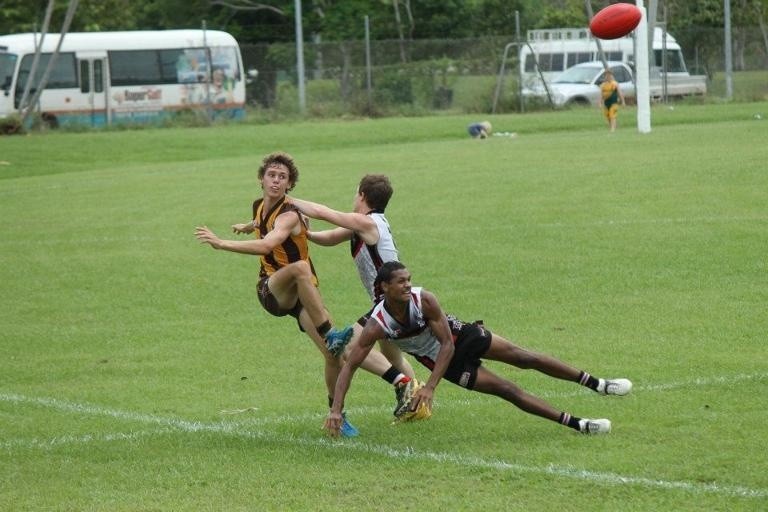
[201,67,232,105]
[320,261,633,437]
[192,151,359,437]
[597,70,626,133]
[283,171,426,418]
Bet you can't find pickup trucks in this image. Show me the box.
[515,57,708,111]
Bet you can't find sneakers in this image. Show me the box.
[391,375,431,425]
[325,325,354,359]
[597,377,632,397]
[575,416,612,435]
[329,408,359,438]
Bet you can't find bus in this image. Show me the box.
[0,26,248,129]
[521,24,691,100]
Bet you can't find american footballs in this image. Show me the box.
[589,2,641,41]
